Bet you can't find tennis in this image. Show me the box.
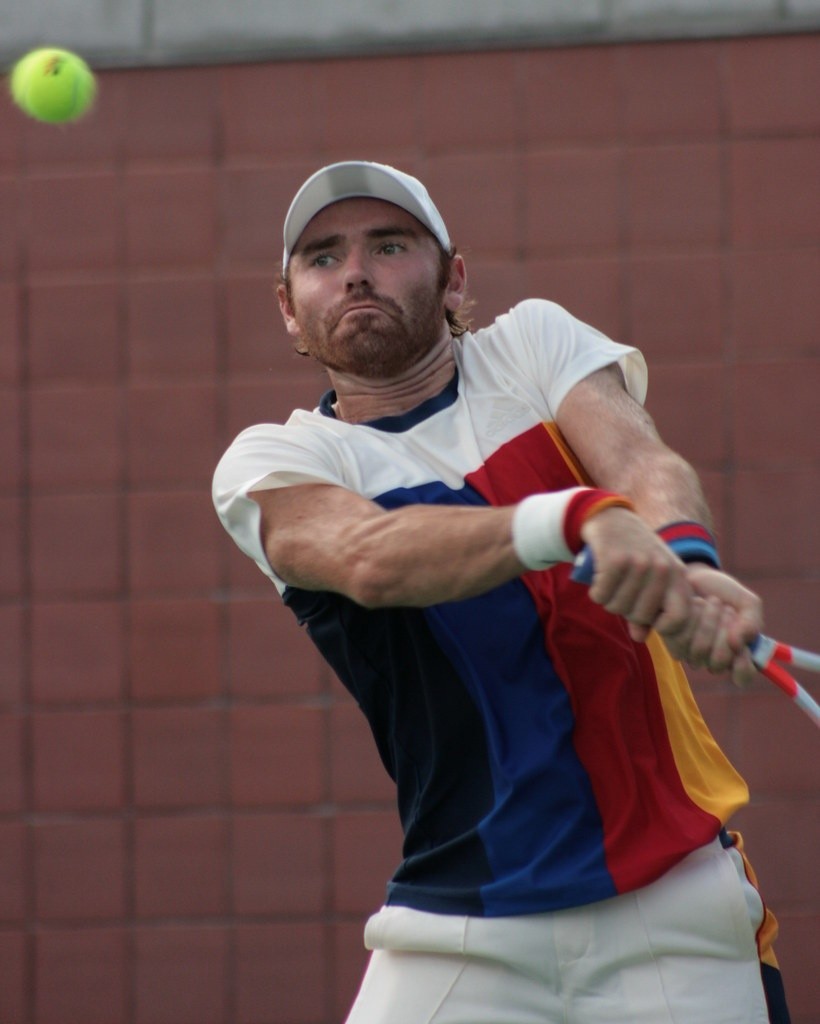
[9,45,98,125]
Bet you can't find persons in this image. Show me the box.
[211,161,790,1024]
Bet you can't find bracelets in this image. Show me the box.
[655,521,721,571]
[512,486,637,571]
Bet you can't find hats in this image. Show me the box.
[280,160,452,274]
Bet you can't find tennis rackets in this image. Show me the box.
[568,540,820,732]
